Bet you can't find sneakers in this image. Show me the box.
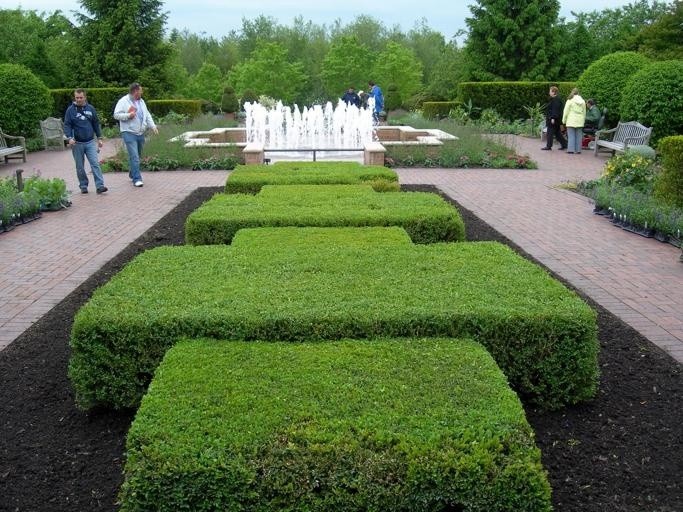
[81,188,88,193]
[130,176,143,186]
[97,186,107,193]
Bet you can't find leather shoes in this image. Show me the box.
[542,145,581,154]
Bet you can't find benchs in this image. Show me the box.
[0,127,26,165]
[39,117,69,152]
[583,108,606,132]
[594,120,653,157]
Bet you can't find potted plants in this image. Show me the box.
[221,87,239,120]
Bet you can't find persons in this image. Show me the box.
[112,82,158,187]
[62,88,108,194]
[561,87,586,154]
[360,79,384,125]
[341,87,359,109]
[540,86,567,150]
[582,99,600,129]
